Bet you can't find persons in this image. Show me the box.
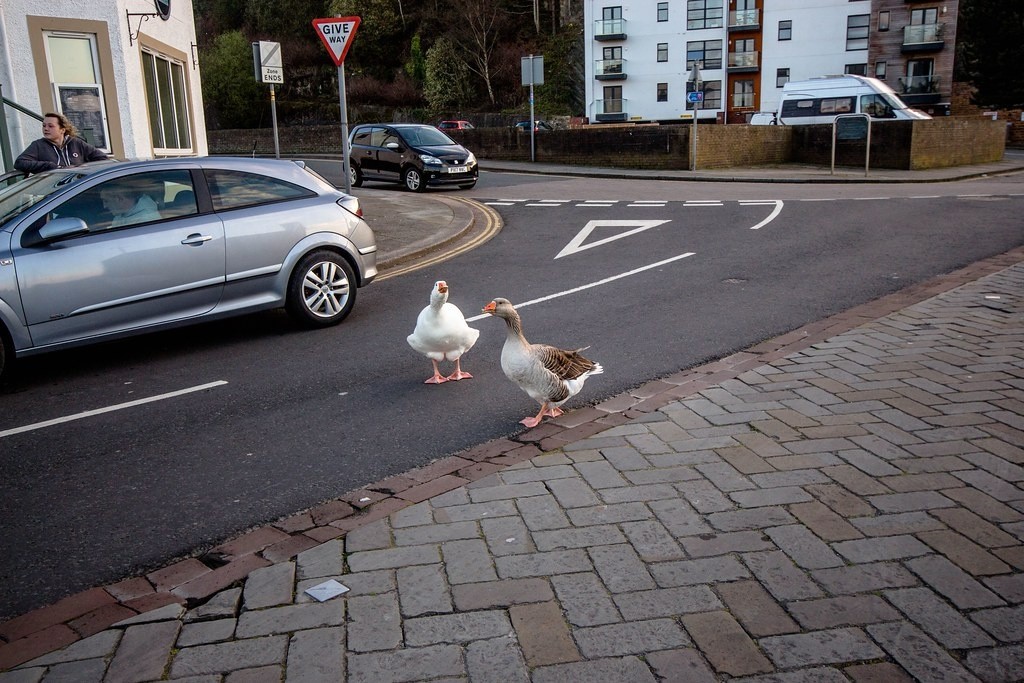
[13,112,111,174]
[101,179,163,228]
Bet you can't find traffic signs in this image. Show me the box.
[687,93,704,104]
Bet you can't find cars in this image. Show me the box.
[438,121,474,130]
[515,121,551,132]
[0,156,379,391]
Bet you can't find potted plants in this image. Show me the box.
[935,23,948,41]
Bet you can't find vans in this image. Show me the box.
[775,74,934,126]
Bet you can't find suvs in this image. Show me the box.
[342,123,477,191]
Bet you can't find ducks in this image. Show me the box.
[484,296,604,427]
[407,280,481,384]
